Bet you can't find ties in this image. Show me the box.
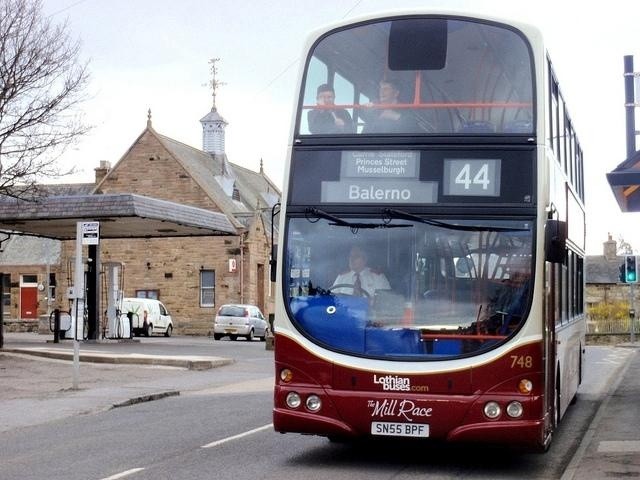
[353,274,362,296]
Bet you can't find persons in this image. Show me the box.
[357,76,424,134]
[307,84,357,136]
[322,243,392,304]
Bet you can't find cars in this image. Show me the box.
[214,304,268,341]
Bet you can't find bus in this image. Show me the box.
[272,7,586,451]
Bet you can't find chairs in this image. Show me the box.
[456,119,532,133]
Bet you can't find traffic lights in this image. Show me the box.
[625,256,637,282]
[618,264,625,282]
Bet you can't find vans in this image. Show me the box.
[116,298,173,337]
